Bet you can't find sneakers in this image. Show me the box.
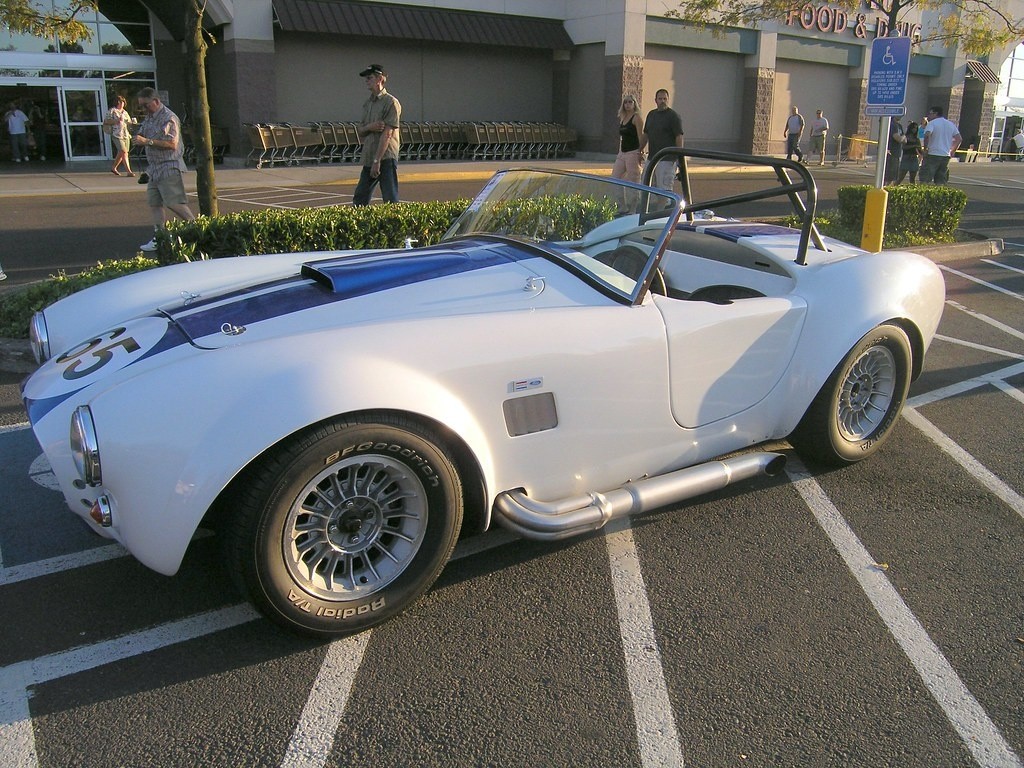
[139,237,158,251]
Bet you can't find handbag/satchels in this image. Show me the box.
[102,119,113,134]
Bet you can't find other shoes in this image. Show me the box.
[126,173,135,176]
[110,169,120,175]
[613,208,636,218]
[802,160,810,165]
[39,156,46,160]
[16,158,22,162]
[24,156,29,161]
[818,162,824,166]
[798,156,804,163]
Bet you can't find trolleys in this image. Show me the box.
[180,103,577,170]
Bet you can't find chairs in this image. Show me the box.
[595,245,768,301]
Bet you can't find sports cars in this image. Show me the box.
[18,146,947,638]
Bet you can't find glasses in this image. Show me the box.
[816,112,820,114]
[33,107,37,109]
[366,76,375,79]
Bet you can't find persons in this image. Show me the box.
[611,94,647,215]
[28,104,48,161]
[897,121,924,185]
[4,102,31,163]
[635,89,685,216]
[131,87,195,252]
[103,96,136,177]
[916,116,929,167]
[803,109,830,166]
[72,105,90,156]
[918,106,963,185]
[1011,128,1024,161]
[783,106,805,163]
[352,64,402,208]
[884,114,907,187]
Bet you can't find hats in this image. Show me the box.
[360,64,385,75]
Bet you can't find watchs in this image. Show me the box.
[373,159,381,164]
[149,139,154,146]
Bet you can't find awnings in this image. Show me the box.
[965,61,1002,84]
